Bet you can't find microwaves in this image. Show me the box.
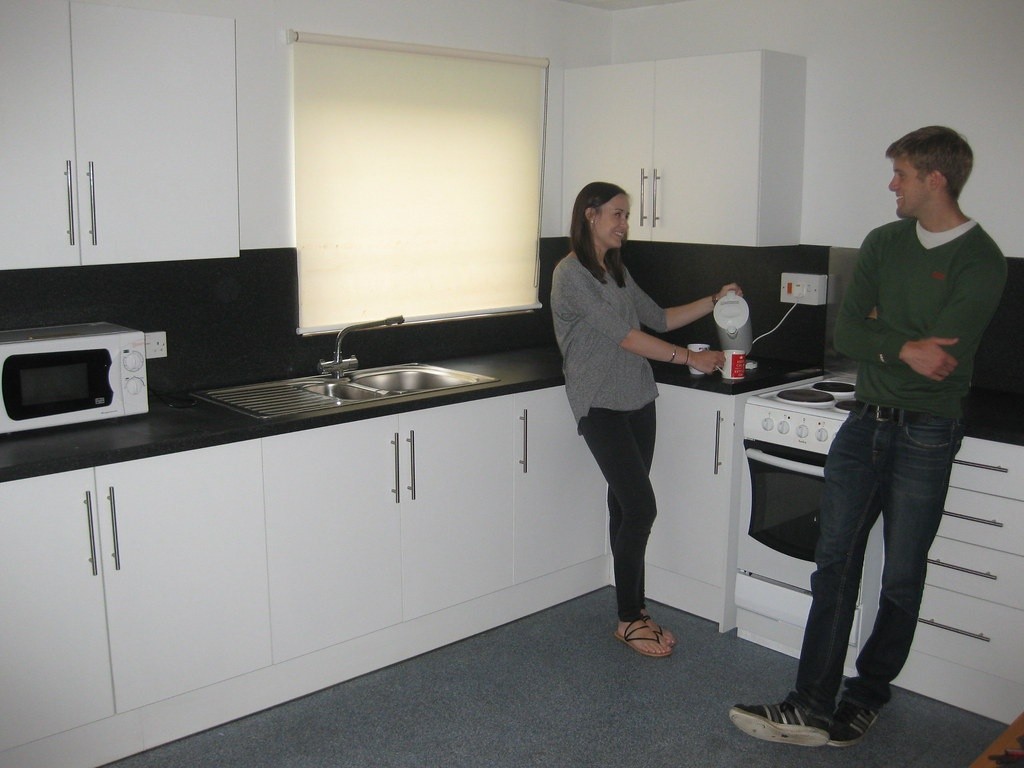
[0,320,150,434]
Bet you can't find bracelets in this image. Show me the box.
[712,293,717,304]
[669,345,676,362]
[684,350,689,365]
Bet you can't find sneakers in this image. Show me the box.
[827,696,886,747]
[728,701,830,747]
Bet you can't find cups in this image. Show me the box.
[713,350,746,379]
[687,344,711,375]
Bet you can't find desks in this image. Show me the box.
[562,48,807,248]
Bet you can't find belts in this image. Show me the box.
[866,405,926,426]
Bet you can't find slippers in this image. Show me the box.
[642,615,674,648]
[614,619,672,657]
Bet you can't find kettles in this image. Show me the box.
[713,290,753,358]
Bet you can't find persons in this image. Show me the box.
[729,125,1007,748]
[550,182,744,658]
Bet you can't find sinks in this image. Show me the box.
[353,369,473,392]
[303,382,383,401]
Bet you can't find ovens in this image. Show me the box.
[737,396,885,679]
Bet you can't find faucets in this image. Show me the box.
[334,316,406,376]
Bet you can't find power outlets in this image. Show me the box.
[780,271,827,307]
[145,331,167,359]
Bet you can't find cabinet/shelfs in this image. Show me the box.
[886,441,1023,724]
[0,441,267,766]
[512,386,611,618]
[0,1,240,272]
[638,386,733,634]
[268,399,513,699]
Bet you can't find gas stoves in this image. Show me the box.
[746,374,859,419]
